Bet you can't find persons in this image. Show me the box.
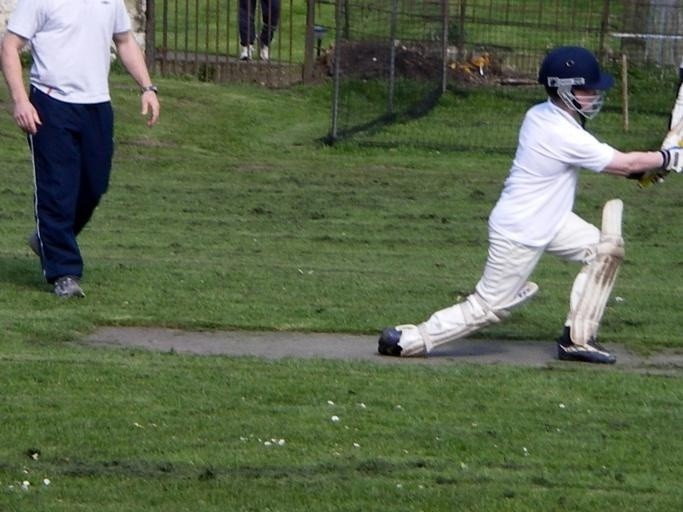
[237,0,281,61]
[375,46,683,364]
[0,0,162,297]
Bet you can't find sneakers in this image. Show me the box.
[554,325,614,363]
[379,327,402,357]
[27,232,84,299]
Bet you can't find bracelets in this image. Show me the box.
[141,85,158,94]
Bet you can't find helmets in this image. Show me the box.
[537,46,612,98]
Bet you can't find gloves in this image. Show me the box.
[625,146,681,186]
